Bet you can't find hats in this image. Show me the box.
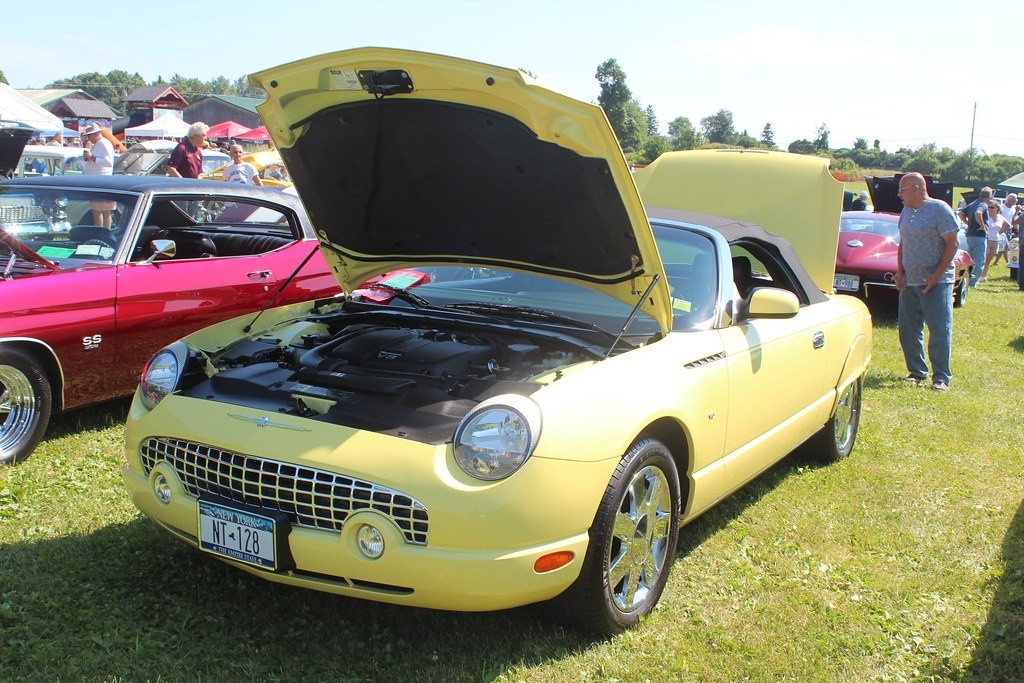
[83,122,105,136]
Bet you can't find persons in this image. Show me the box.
[167,122,210,220]
[958,186,1024,292]
[269,165,290,182]
[894,173,960,391]
[127,137,236,155]
[83,125,116,230]
[223,144,263,207]
[28,122,111,176]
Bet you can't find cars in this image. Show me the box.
[831,172,975,308]
[1005,238,1019,281]
[0,176,345,472]
[59,140,230,229]
[957,191,1009,255]
[122,40,876,638]
[0,126,57,244]
[201,151,296,190]
[14,145,120,185]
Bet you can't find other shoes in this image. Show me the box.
[932,380,947,390]
[905,374,925,382]
[979,276,986,281]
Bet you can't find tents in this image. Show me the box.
[0,81,79,146]
[125,112,191,148]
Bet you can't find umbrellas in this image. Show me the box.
[207,121,272,140]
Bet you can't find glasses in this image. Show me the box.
[899,185,915,192]
[988,206,995,209]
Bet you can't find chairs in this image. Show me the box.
[66,226,118,258]
[693,253,751,300]
[162,232,217,260]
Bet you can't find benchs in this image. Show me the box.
[148,229,289,257]
